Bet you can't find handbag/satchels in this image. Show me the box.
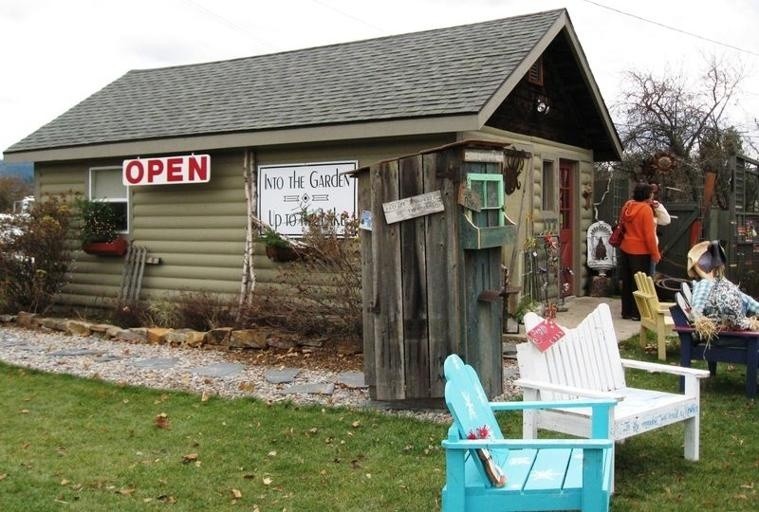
[608,224,624,247]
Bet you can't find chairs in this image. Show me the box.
[515,302,711,494]
[632,271,677,361]
[669,279,759,398]
[440,353,618,512]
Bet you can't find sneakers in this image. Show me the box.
[623,314,640,321]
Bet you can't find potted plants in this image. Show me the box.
[256,226,289,261]
[76,195,127,257]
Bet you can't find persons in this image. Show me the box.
[647,200,671,276]
[619,183,662,321]
